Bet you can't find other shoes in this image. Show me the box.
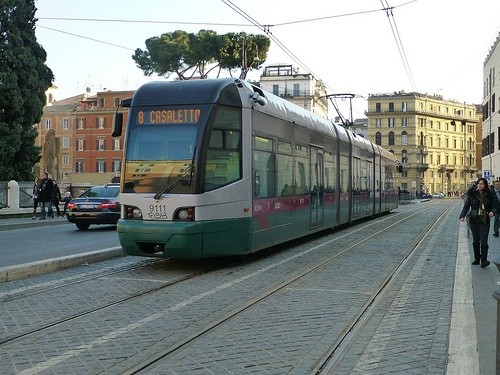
[60,212,64,217]
[39,216,45,220]
[493,232,499,237]
[472,260,480,265]
[481,261,490,268]
[51,213,55,219]
[31,216,36,220]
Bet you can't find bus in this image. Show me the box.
[110,77,399,260]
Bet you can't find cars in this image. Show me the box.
[65,185,121,230]
[432,193,444,198]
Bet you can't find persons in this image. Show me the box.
[416,189,424,198]
[31,172,61,220]
[463,185,474,217]
[60,191,71,217]
[76,190,88,198]
[280,184,380,208]
[459,178,500,270]
[448,191,458,197]
[488,181,500,237]
